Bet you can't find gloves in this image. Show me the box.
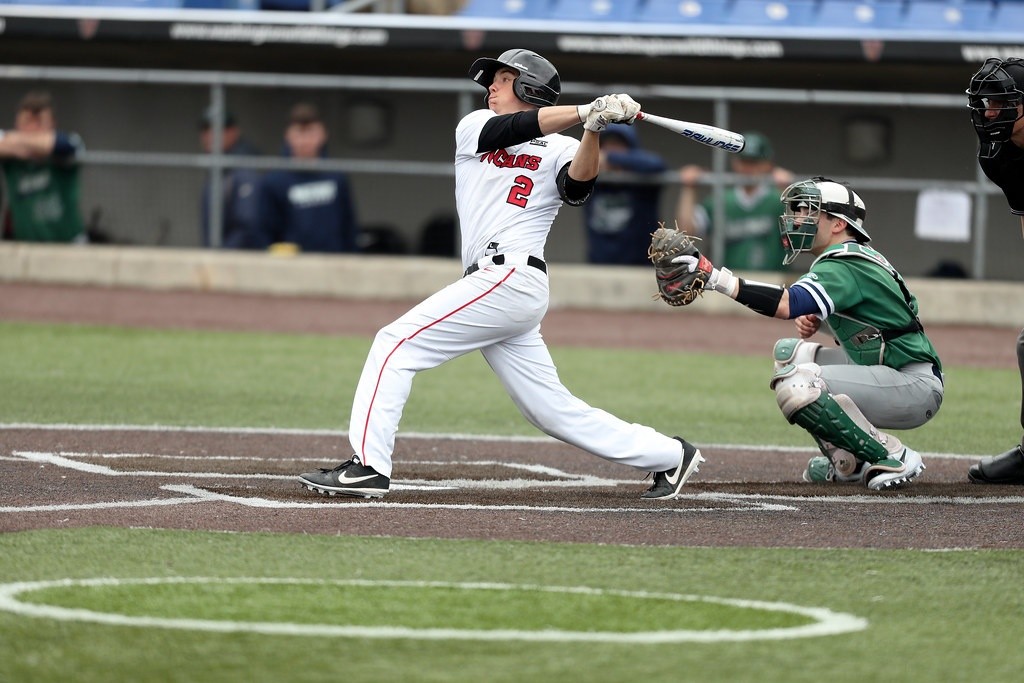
[671,255,737,297]
[577,91,642,133]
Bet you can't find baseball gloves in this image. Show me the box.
[650,224,712,309]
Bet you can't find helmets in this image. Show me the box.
[467,49,563,108]
[795,176,873,242]
[974,57,1024,102]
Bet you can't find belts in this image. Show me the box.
[464,253,547,277]
[931,363,944,388]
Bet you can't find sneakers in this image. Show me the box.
[642,436,706,499]
[807,455,873,483]
[866,446,927,491]
[297,454,390,500]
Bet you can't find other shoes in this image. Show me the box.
[967,462,987,484]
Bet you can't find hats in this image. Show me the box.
[196,102,237,130]
[728,136,765,163]
[595,116,640,149]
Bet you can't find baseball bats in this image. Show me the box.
[593,98,745,154]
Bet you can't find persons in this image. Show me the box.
[580,122,668,267]
[648,173,945,493]
[677,132,798,272]
[199,103,265,249]
[0,87,90,243]
[296,48,705,500]
[258,101,357,254]
[965,56,1024,485]
